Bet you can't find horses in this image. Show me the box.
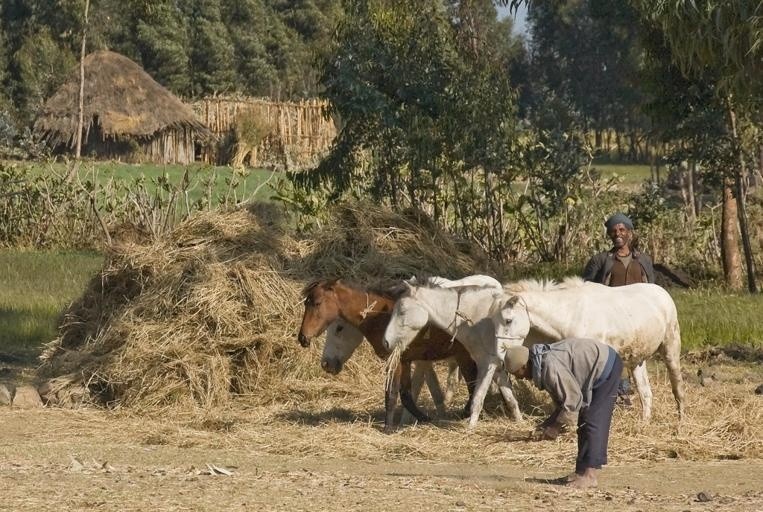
[486,274,686,426]
[320,274,504,427]
[297,275,478,423]
[381,270,613,432]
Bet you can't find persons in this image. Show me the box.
[505,336,623,489]
[583,212,654,406]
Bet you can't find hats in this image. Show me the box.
[505,346,529,373]
[605,214,633,232]
